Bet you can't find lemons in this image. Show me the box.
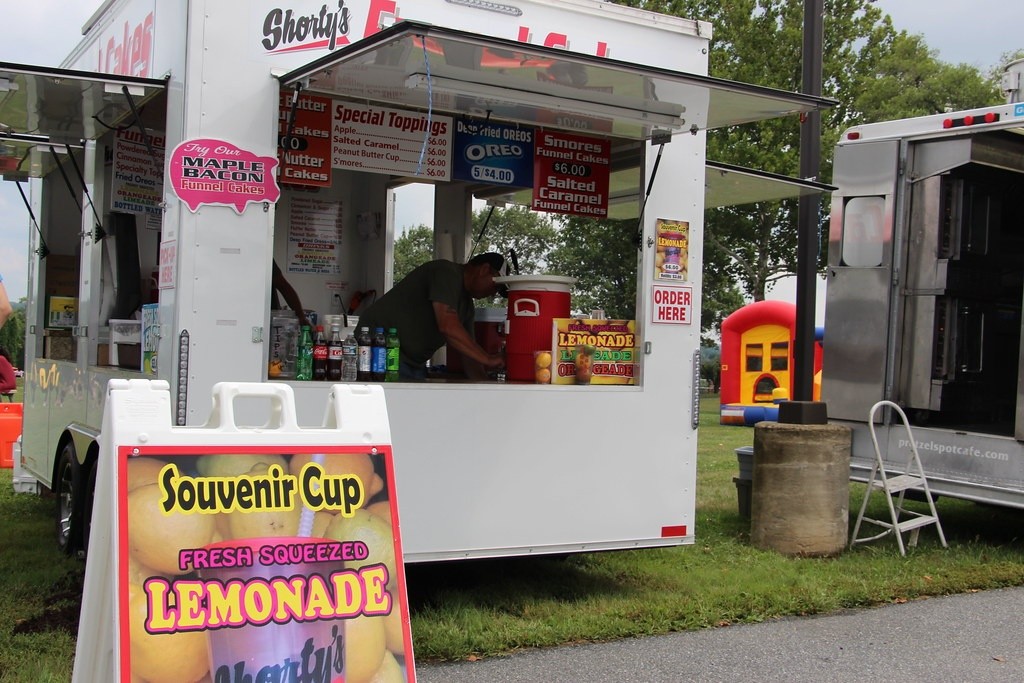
[534,353,552,385]
[575,346,596,384]
[125,452,410,682]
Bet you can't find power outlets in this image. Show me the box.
[331,291,341,307]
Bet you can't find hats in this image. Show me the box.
[485,252,510,298]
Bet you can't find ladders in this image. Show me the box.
[850,399,949,558]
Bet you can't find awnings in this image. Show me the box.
[466,160,839,265]
[274,18,841,230]
[0,60,167,255]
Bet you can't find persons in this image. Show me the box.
[0,275,17,401]
[355,252,509,376]
[272,259,318,340]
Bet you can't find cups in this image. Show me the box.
[190,536,350,683]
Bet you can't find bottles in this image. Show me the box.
[358,327,371,381]
[386,328,399,382]
[327,328,342,381]
[342,332,358,381]
[313,326,327,380]
[372,328,386,382]
[296,326,312,380]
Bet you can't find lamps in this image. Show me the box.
[404,74,686,130]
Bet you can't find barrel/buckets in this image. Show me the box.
[493,275,573,380]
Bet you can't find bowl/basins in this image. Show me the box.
[114,341,142,370]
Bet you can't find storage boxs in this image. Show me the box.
[446,307,507,365]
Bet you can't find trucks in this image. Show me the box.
[0,1,847,569]
[819,99,1024,514]
